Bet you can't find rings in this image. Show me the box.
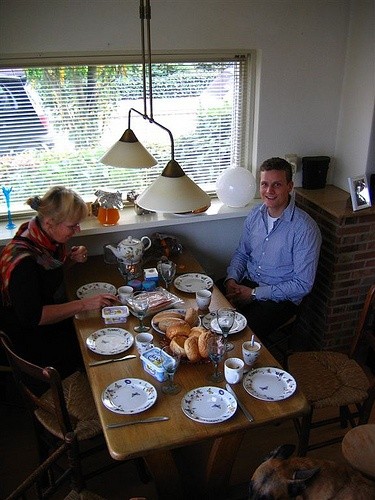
[83,255,86,259]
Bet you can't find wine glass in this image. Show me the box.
[159,307,237,394]
[131,260,178,331]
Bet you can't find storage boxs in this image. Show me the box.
[140,347,176,383]
[102,306,129,324]
[144,268,159,283]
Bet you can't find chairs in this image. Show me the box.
[275,284,375,457]
[0,332,152,500]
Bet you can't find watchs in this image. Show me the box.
[251,288,256,303]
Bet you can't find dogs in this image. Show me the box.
[244,441,375,500]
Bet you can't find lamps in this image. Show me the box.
[100,0,211,213]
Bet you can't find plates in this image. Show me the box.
[152,272,247,335]
[181,385,237,423]
[76,282,135,355]
[243,367,297,402]
[101,378,158,413]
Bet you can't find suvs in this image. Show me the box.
[0,73,54,152]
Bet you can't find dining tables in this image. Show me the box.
[65,248,311,500]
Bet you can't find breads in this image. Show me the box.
[152,307,215,362]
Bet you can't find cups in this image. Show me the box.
[135,332,155,355]
[196,289,212,311]
[98,206,120,226]
[223,340,262,384]
[116,285,134,305]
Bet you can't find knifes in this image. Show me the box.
[106,416,169,427]
[226,382,254,423]
[88,354,137,366]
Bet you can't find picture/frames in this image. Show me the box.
[348,174,371,211]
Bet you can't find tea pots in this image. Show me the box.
[105,235,152,265]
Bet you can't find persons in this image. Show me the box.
[217,157,322,347]
[0,185,118,380]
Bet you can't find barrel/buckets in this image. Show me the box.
[302,156,330,190]
